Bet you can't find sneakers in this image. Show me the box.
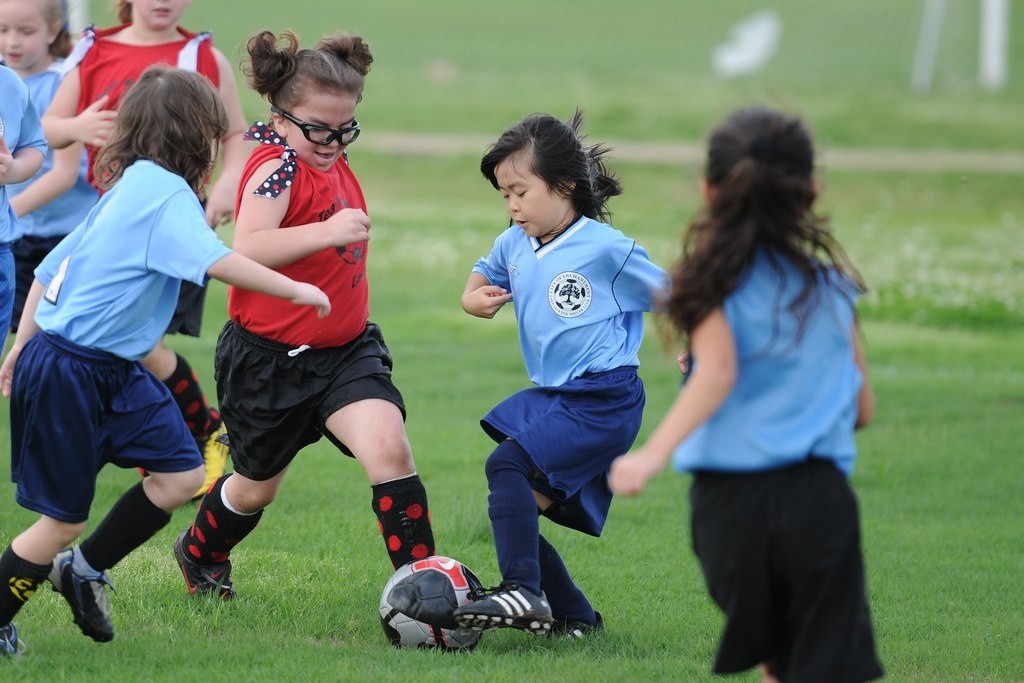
[47,547,118,641]
[172,528,237,600]
[453,581,554,637]
[0,622,28,660]
[547,611,604,641]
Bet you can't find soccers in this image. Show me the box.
[378,555,488,656]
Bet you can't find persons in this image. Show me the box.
[41,1,252,503]
[172,30,437,614]
[0,0,102,354]
[454,105,674,640]
[608,106,887,683]
[0,66,331,657]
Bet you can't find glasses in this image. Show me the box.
[270,105,361,145]
